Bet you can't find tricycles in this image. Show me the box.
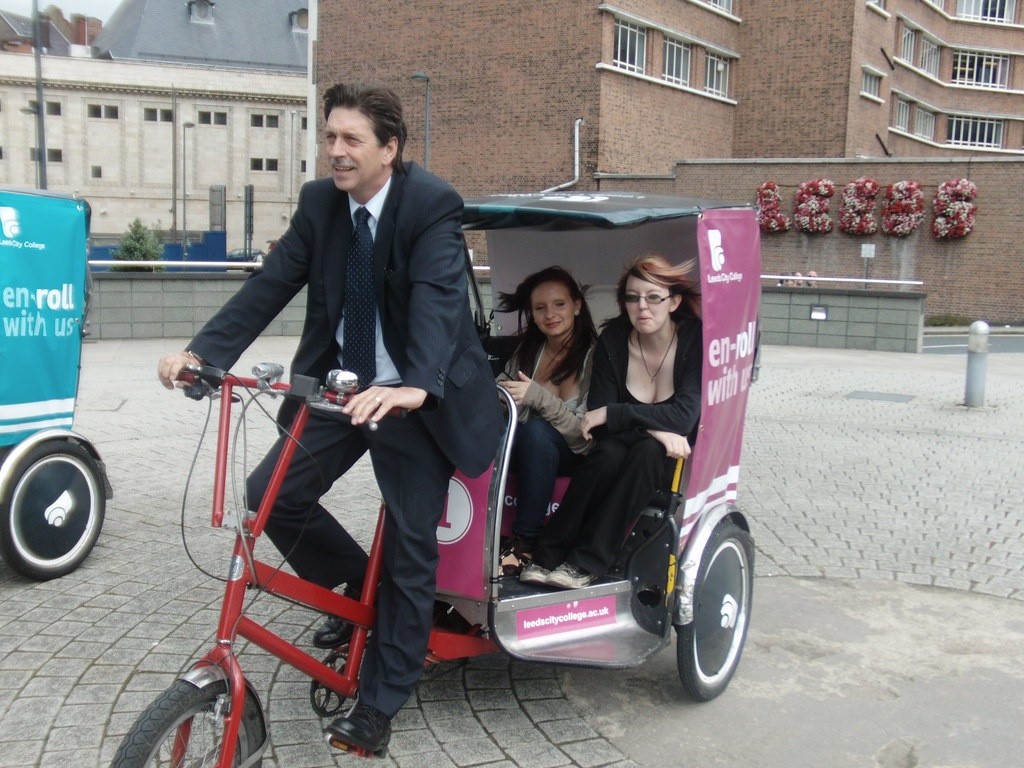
[0,191,113,581]
[109,194,760,767]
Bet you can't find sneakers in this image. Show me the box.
[519,563,550,584]
[545,562,598,589]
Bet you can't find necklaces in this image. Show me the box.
[637,323,677,383]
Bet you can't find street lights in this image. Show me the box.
[410,72,429,171]
[183,121,195,271]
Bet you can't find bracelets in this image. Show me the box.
[183,349,205,364]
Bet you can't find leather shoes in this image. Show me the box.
[326,705,391,758]
[313,584,364,648]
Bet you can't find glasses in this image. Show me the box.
[622,292,671,304]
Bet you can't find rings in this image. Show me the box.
[374,396,382,404]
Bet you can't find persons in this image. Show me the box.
[519,254,704,589]
[493,265,597,574]
[157,84,507,759]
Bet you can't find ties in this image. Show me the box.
[341,206,376,390]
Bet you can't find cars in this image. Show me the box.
[226,248,267,270]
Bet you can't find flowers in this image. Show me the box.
[754,179,979,242]
